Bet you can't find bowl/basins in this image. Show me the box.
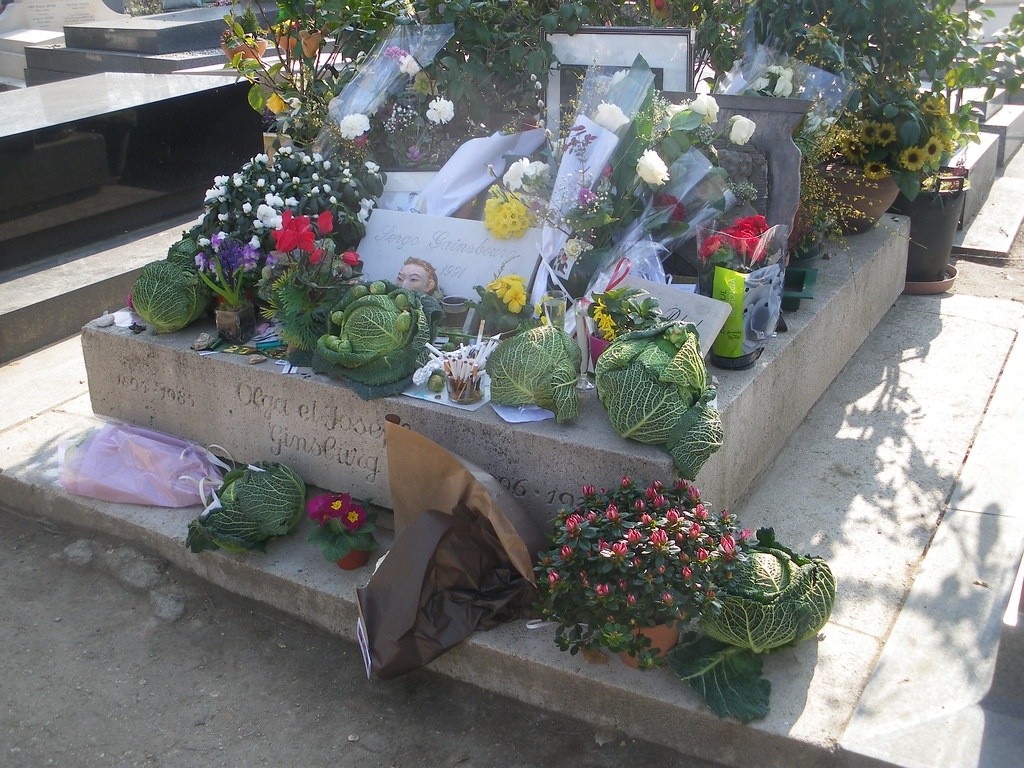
[590,333,611,370]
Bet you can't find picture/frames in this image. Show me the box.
[541,27,694,134]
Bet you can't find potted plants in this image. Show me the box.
[905,0,1024,293]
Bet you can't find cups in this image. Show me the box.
[446,375,485,405]
[440,295,469,327]
[541,291,567,331]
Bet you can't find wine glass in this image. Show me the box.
[572,297,596,389]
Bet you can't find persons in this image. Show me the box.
[396,257,438,296]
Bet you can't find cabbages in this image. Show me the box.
[186,462,306,553]
[130,238,215,334]
[485,323,583,423]
[594,321,724,479]
[311,281,446,403]
[663,528,835,720]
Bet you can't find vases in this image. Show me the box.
[824,165,902,235]
[335,542,373,568]
[605,611,679,674]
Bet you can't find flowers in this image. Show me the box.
[312,491,382,561]
[487,81,783,361]
[194,37,443,321]
[798,24,963,182]
[530,474,749,671]
